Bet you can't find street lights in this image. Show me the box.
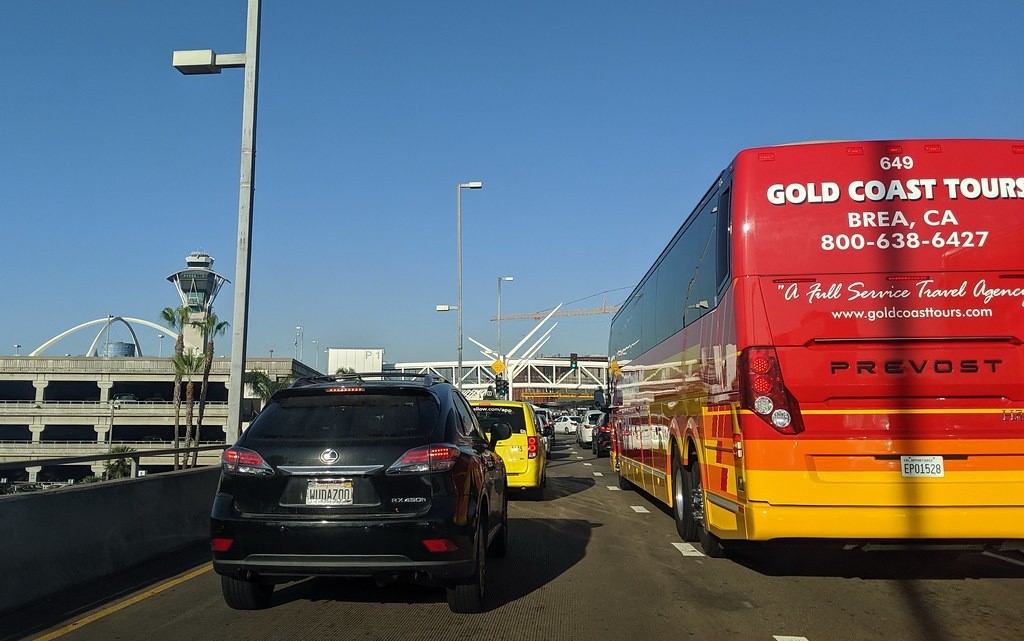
[173,46,259,443]
[13,344,21,355]
[158,334,164,357]
[435,306,462,394]
[312,340,319,370]
[106,315,114,357]
[270,349,273,358]
[497,276,515,355]
[296,326,304,362]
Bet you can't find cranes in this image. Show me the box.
[490,292,623,321]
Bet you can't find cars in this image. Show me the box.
[144,397,166,404]
[553,416,584,435]
[112,393,138,404]
[0,484,43,493]
[535,408,555,457]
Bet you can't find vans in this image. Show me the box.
[467,401,546,492]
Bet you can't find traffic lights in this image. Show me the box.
[496,375,501,394]
[501,381,509,396]
[571,354,577,369]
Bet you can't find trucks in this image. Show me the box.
[101,341,135,357]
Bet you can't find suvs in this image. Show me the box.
[211,370,509,611]
[575,410,602,449]
[592,412,611,458]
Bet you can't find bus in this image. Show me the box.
[35,481,68,490]
[594,138,1024,550]
